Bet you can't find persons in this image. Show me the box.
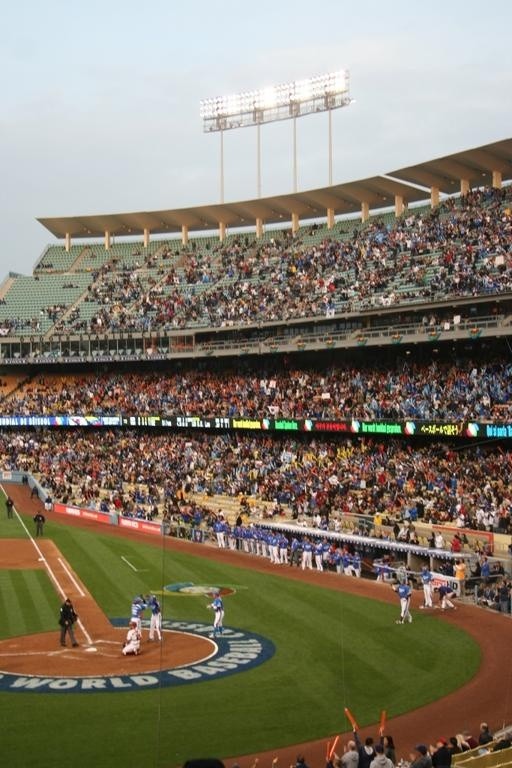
[128,593,147,631]
[147,595,163,645]
[205,591,225,637]
[2,185,512,627]
[122,621,142,657]
[230,719,511,767]
[57,596,79,647]
